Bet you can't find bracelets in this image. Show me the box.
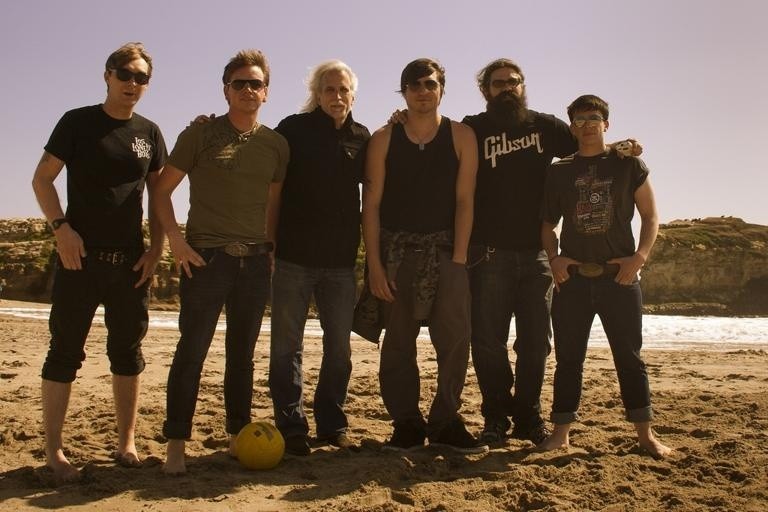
[548,255,557,266]
[166,228,184,241]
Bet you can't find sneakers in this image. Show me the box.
[285,432,313,458]
[513,422,550,444]
[427,424,491,453]
[326,429,358,448]
[479,422,507,444]
[382,422,427,453]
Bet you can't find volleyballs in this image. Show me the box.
[235,422,284,470]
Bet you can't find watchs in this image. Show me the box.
[50,217,67,231]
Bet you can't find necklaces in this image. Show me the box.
[224,112,260,145]
[410,119,436,151]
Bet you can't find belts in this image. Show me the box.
[86,248,134,266]
[565,262,620,279]
[211,242,272,258]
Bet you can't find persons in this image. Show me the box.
[185,59,408,457]
[540,92,672,466]
[31,41,169,483]
[387,57,644,445]
[360,57,490,455]
[148,46,291,479]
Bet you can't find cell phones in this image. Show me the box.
[615,141,634,157]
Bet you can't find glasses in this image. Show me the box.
[109,67,149,85]
[489,79,523,89]
[227,80,265,90]
[572,117,607,129]
[404,80,438,92]
